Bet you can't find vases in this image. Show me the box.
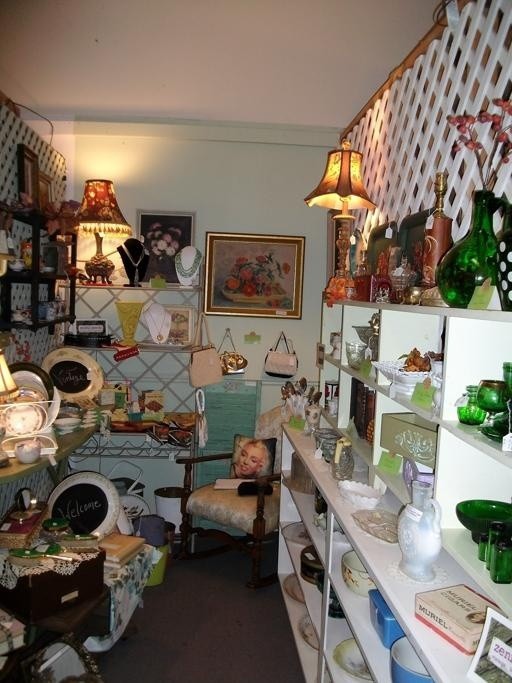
[431,190,504,307]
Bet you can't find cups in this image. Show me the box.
[304,404,323,435]
[313,427,341,461]
[11,308,29,322]
[327,395,339,416]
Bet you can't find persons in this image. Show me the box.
[236,439,267,478]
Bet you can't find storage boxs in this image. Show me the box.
[0,544,107,621]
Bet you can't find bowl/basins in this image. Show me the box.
[14,440,43,465]
[389,636,432,682]
[52,415,82,430]
[457,499,512,542]
[9,257,26,272]
[341,549,376,595]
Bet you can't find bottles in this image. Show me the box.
[356,249,370,276]
[36,299,67,322]
[502,362,512,389]
[455,385,485,425]
[330,435,357,480]
[479,521,512,585]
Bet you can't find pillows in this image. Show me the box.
[228,434,278,479]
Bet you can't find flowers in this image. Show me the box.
[147,222,182,258]
[222,245,291,299]
[447,97,512,194]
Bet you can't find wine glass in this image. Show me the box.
[477,379,509,440]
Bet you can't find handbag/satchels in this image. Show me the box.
[188,311,223,388]
[216,326,249,376]
[264,330,299,380]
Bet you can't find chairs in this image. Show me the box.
[175,403,310,587]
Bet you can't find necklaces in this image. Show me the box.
[146,307,166,341]
[174,246,202,279]
[122,243,146,287]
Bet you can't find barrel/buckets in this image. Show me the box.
[163,520,176,567]
[133,514,164,546]
[143,538,169,587]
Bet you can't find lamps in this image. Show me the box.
[0,349,22,467]
[306,139,377,297]
[75,179,134,284]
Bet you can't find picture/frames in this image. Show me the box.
[135,206,196,283]
[39,169,56,207]
[16,142,41,207]
[46,469,119,539]
[203,230,305,319]
[161,304,195,343]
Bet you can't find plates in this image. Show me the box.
[350,508,400,543]
[295,613,322,648]
[42,267,56,273]
[284,572,303,605]
[331,636,371,680]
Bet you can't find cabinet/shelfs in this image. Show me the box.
[1,208,77,332]
[312,292,512,651]
[274,420,511,683]
[36,587,113,652]
[58,284,202,547]
